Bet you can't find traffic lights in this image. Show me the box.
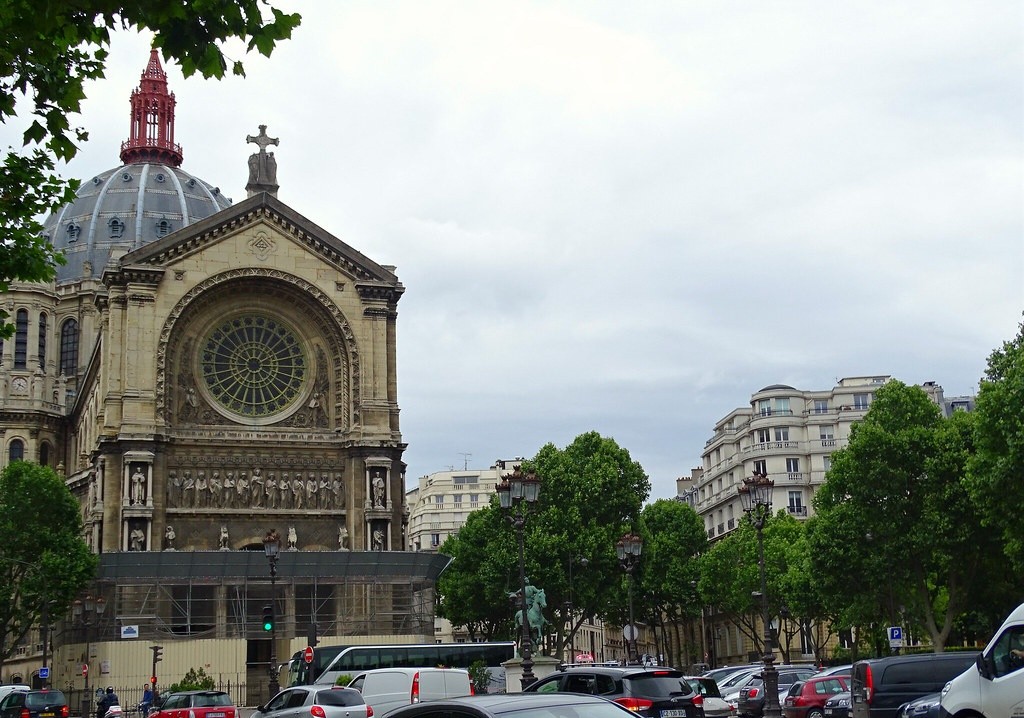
[151,676,157,683]
[153,645,163,663]
[263,615,272,631]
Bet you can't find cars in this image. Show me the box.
[248,684,376,718]
[147,689,241,718]
[899,691,943,718]
[682,663,855,718]
[380,692,644,718]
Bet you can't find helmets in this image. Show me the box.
[106,687,113,694]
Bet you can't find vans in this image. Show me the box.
[939,603,1024,718]
[0,685,32,711]
[346,666,474,718]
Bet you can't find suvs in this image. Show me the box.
[850,649,985,718]
[524,662,705,718]
[0,688,69,718]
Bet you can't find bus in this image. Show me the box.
[276,641,517,718]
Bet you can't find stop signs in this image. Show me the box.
[305,646,314,664]
[82,664,88,678]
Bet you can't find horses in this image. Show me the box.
[514,588,552,658]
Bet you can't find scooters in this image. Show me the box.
[95,699,123,718]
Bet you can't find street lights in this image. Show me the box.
[74,595,107,718]
[569,552,588,668]
[494,464,545,693]
[616,532,643,666]
[261,527,283,699]
[736,468,785,717]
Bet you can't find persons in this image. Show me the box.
[1012,649,1024,657]
[373,526,386,549]
[167,469,344,510]
[130,523,145,551]
[372,472,385,506]
[646,654,652,665]
[95,687,119,718]
[142,684,153,718]
[132,468,145,504]
[515,576,540,608]
